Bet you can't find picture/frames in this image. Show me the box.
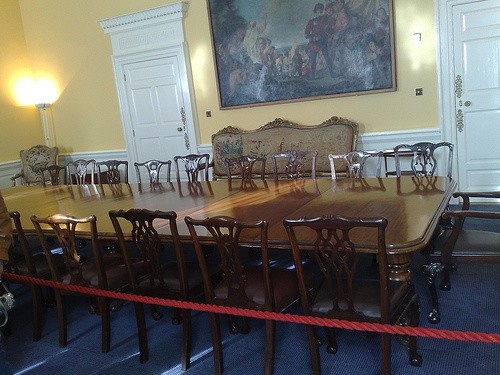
[206,0,397,111]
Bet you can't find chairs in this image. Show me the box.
[0,145,500,375]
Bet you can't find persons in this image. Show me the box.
[0,193,12,277]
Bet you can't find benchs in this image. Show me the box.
[212,116,359,179]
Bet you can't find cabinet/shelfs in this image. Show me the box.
[383,151,427,177]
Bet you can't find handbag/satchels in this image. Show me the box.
[0,280,16,328]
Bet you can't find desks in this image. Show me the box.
[0,176,457,351]
